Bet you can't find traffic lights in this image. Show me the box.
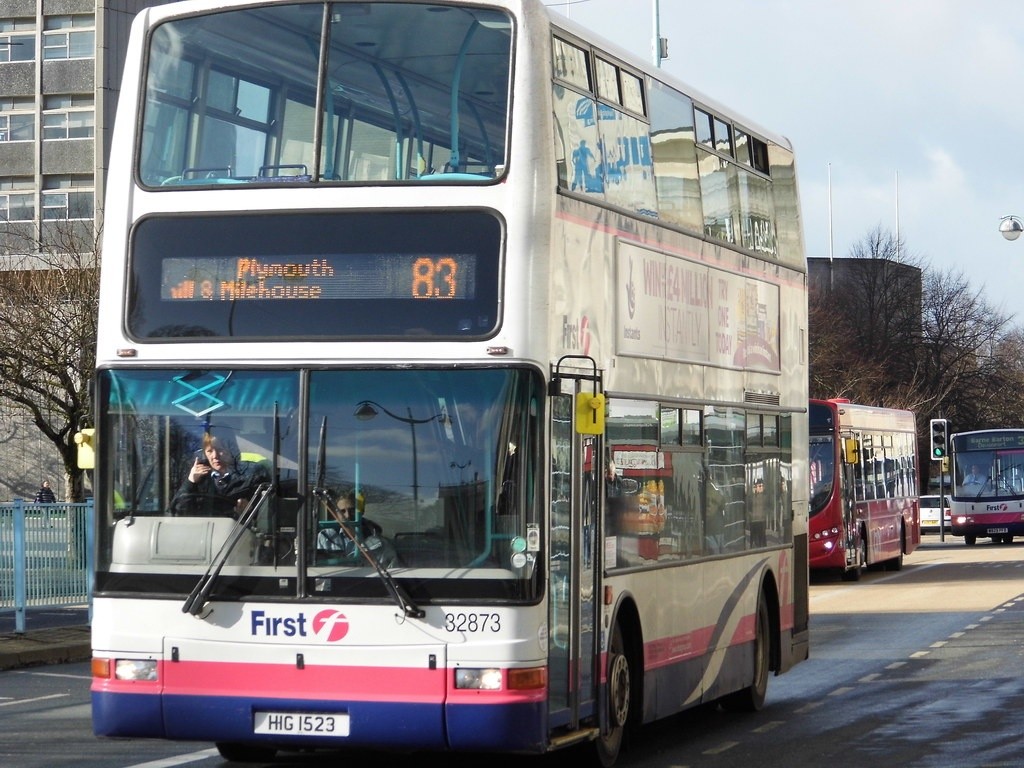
[930,419,948,461]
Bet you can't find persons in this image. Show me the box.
[962,464,987,486]
[169,423,272,528]
[704,479,725,554]
[571,140,596,191]
[86,469,126,511]
[775,470,791,544]
[316,492,410,600]
[36,479,56,529]
[749,478,768,548]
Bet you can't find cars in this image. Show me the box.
[917,494,952,536]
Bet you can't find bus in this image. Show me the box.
[809,396,921,580]
[72,0,812,768]
[940,428,1024,545]
